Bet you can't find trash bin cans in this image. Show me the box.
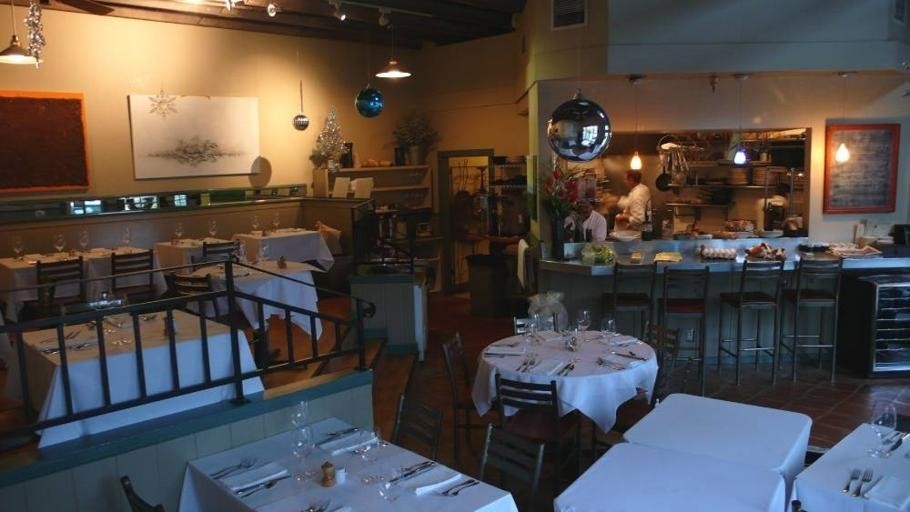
[465,253,514,315]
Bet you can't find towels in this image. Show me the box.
[517,239,530,288]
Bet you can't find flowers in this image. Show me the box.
[540,162,608,213]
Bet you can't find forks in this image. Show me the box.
[840,468,860,494]
[850,468,873,498]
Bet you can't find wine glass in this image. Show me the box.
[866,400,897,460]
[78,230,90,255]
[206,218,218,242]
[289,425,316,489]
[173,222,185,245]
[357,425,383,488]
[575,309,592,342]
[288,399,311,429]
[524,322,538,355]
[52,229,67,259]
[376,461,405,512]
[123,225,137,254]
[527,310,543,345]
[250,214,262,236]
[601,317,618,356]
[11,234,25,262]
[272,212,281,234]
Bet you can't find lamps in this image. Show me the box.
[375,22,412,79]
[832,71,857,165]
[0,0,42,65]
[378,7,391,25]
[267,0,282,17]
[730,73,750,165]
[333,2,346,20]
[625,74,647,170]
[659,134,681,150]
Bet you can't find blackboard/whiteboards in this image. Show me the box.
[0,90,94,192]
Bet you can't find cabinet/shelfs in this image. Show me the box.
[313,165,444,294]
[857,274,910,374]
[474,164,526,244]
[666,135,808,191]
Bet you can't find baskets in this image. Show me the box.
[727,220,754,232]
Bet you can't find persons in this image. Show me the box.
[563,201,608,242]
[605,169,652,224]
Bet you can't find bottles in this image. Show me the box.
[334,466,346,486]
[320,461,336,488]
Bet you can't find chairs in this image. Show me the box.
[476,422,547,512]
[601,260,658,341]
[781,255,844,384]
[22,254,86,321]
[103,248,154,305]
[165,271,221,323]
[120,475,166,512]
[511,311,560,335]
[439,330,499,460]
[391,394,444,462]
[202,239,241,263]
[660,264,710,399]
[494,374,578,499]
[716,258,785,386]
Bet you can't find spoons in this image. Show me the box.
[238,478,278,500]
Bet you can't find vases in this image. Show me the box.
[556,206,584,261]
[394,147,406,166]
[408,142,422,165]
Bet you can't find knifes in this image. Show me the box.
[886,432,910,455]
